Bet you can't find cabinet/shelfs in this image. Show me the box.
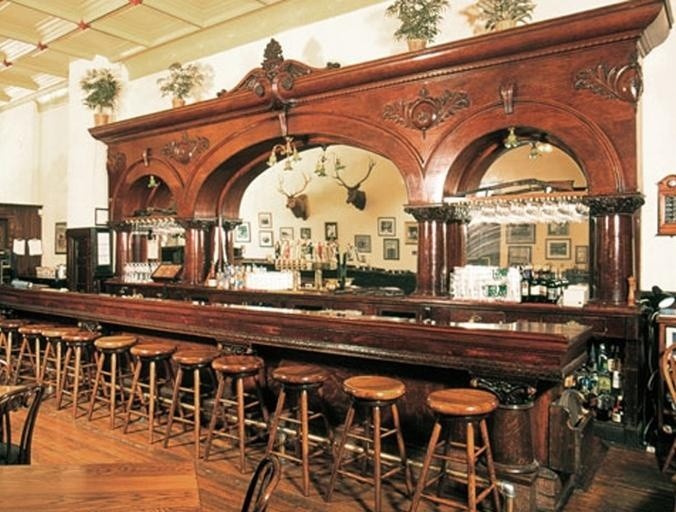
[66,229,97,294]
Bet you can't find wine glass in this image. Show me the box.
[463,199,592,223]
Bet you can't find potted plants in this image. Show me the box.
[380,1,450,54]
[458,0,537,37]
[153,64,205,110]
[80,64,121,126]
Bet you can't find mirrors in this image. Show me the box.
[219,144,419,282]
[461,143,591,274]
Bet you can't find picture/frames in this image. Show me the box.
[95,208,110,226]
[505,221,590,268]
[54,221,66,255]
[229,214,420,262]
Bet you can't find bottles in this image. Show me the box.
[203,237,362,296]
[119,216,186,287]
[445,261,571,304]
[33,262,69,283]
[571,341,627,422]
[626,272,638,308]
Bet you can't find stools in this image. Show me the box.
[1,318,107,418]
[410,388,501,512]
[86,334,148,428]
[266,362,334,496]
[203,353,270,475]
[324,374,415,511]
[164,347,217,459]
[121,341,188,444]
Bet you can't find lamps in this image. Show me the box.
[637,284,676,454]
[145,174,163,190]
[311,141,350,179]
[502,126,522,149]
[265,133,303,173]
[527,141,543,160]
